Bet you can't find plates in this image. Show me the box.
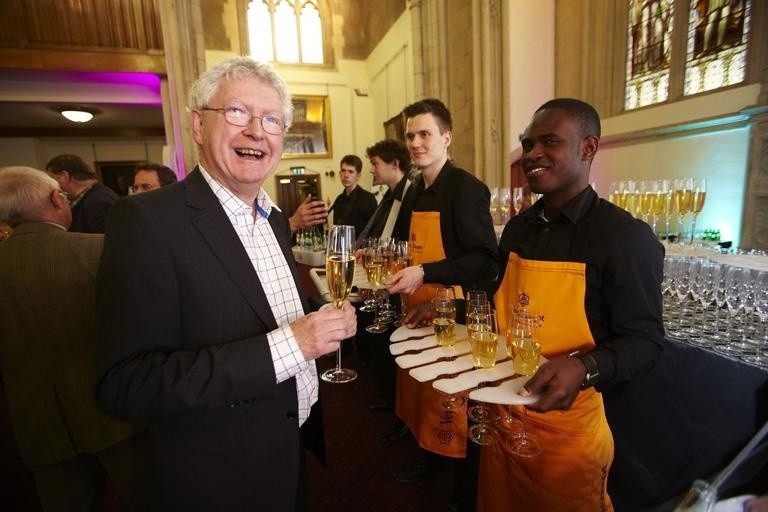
[715,493,759,512]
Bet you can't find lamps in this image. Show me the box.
[58,106,95,125]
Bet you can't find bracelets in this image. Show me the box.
[568,350,601,389]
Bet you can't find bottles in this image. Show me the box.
[702,229,720,241]
[295,231,314,251]
[674,479,720,512]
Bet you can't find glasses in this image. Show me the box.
[202,106,288,135]
[59,191,73,205]
[132,183,159,193]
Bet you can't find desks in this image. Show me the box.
[603,291,768,512]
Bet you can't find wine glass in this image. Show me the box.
[319,222,361,385]
[357,234,415,336]
[660,254,768,369]
[489,177,708,251]
[429,286,546,459]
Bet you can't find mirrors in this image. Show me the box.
[275,93,334,160]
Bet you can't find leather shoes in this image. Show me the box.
[367,394,475,511]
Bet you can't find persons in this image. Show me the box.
[385,99,499,512]
[288,191,328,235]
[327,155,378,238]
[345,140,413,259]
[133,162,177,193]
[87,58,358,512]
[397,98,664,512]
[46,154,120,235]
[0,165,158,512]
